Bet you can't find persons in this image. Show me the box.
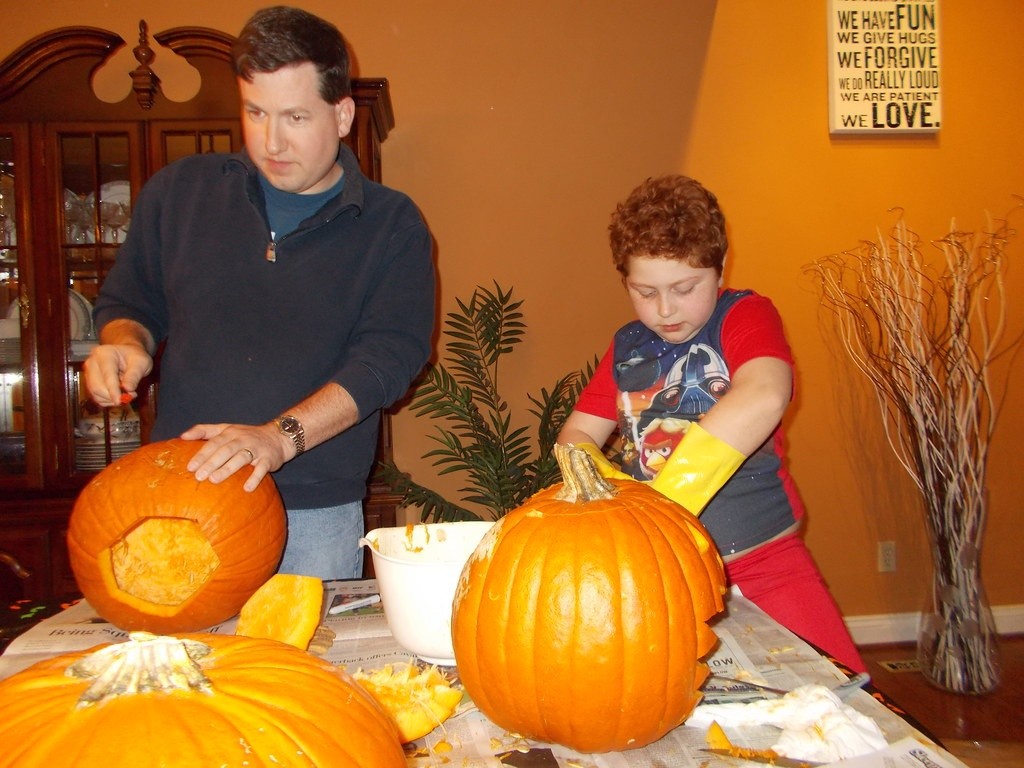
[82,5,436,581]
[556,174,873,683]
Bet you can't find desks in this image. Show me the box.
[2,576,949,768]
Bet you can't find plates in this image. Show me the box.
[0,170,16,223]
[63,187,82,244]
[73,439,141,470]
[5,288,95,340]
[85,181,130,243]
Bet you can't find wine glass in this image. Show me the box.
[0,194,132,246]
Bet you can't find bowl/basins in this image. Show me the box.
[359,520,497,667]
[0,433,28,474]
[77,419,138,441]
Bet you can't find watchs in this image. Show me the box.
[274,414,305,455]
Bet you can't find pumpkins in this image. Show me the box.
[0,437,728,768]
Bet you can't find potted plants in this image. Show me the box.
[801,189,1024,695]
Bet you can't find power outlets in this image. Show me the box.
[877,540,896,572]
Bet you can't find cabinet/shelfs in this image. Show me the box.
[1,20,396,653]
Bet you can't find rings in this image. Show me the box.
[243,449,253,461]
